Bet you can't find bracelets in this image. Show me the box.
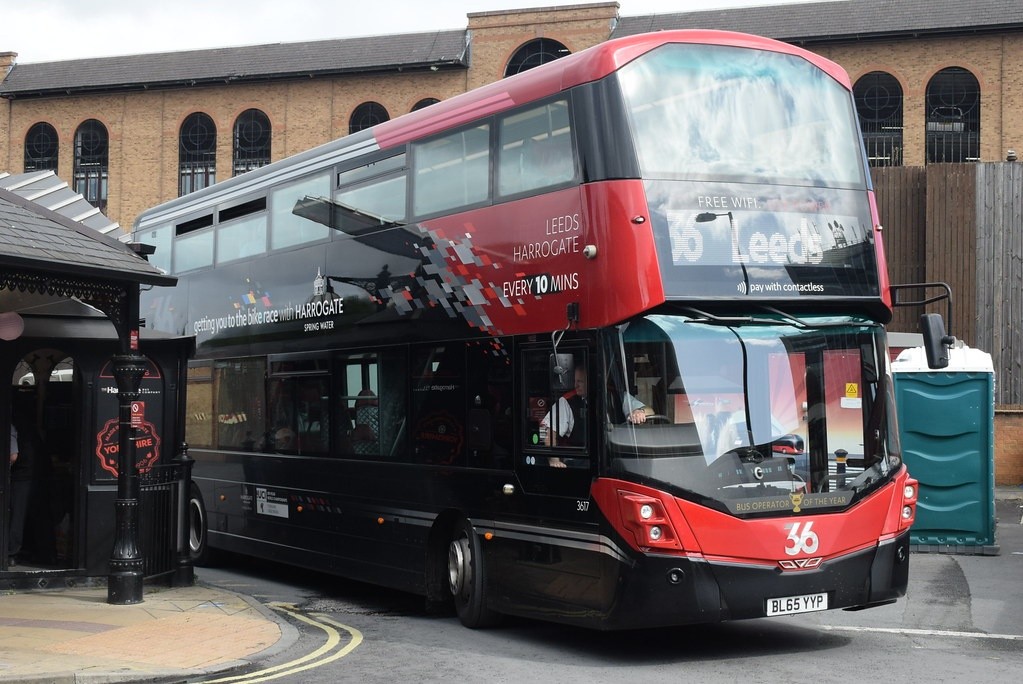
[640,408,646,414]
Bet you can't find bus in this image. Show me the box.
[131,27,959,628]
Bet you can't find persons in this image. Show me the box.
[8,391,37,568]
[542,361,655,468]
